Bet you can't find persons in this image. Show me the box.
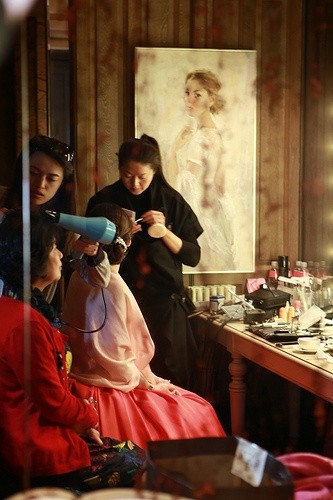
[0,133,228,500]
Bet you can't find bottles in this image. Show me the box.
[269,255,307,288]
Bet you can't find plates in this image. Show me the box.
[297,348,321,354]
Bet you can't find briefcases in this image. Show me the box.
[245,282,291,322]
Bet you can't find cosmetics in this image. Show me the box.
[268,256,322,290]
[279,300,326,333]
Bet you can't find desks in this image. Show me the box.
[195,308,333,458]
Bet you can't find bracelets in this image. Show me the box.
[147,381,153,391]
[81,426,95,435]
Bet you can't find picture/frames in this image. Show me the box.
[134,46,258,275]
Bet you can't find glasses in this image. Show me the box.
[34,136,74,162]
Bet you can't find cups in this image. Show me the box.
[298,337,321,350]
